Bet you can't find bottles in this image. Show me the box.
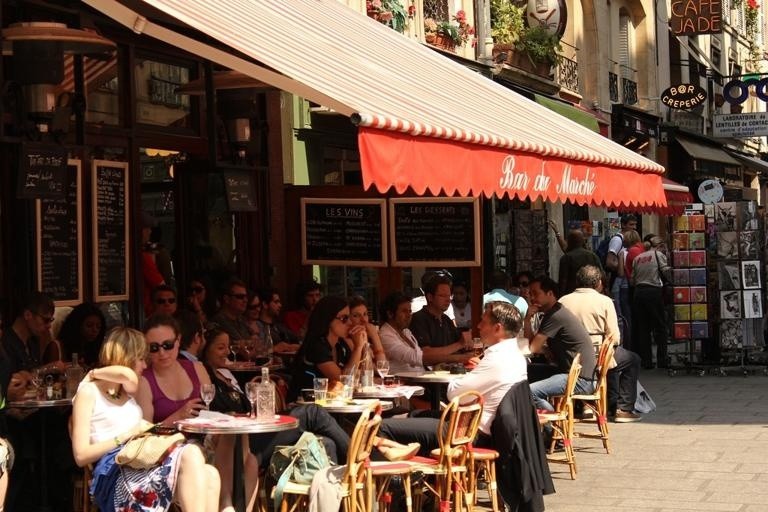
[262,324,273,365]
[67,352,83,398]
[361,342,373,391]
[257,365,276,422]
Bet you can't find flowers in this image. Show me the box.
[744,1,761,75]
[425,9,479,50]
[366,1,416,33]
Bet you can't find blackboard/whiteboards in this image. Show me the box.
[301,197,388,268]
[90,159,130,303]
[389,197,480,267]
[222,172,258,212]
[17,140,68,199]
[35,158,84,307]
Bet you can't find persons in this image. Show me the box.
[547,215,669,368]
[139,213,166,319]
[0,265,643,512]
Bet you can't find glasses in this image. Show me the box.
[251,303,262,310]
[149,337,177,353]
[39,314,55,324]
[156,297,177,304]
[517,282,529,288]
[335,314,349,324]
[194,286,205,294]
[233,293,247,300]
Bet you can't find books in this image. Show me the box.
[671,200,767,364]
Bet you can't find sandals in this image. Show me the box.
[376,437,421,462]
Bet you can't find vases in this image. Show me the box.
[368,9,381,20]
[434,32,455,54]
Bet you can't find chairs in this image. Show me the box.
[69,303,618,511]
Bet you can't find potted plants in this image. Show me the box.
[490,1,557,81]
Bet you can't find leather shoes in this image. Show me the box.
[387,471,424,492]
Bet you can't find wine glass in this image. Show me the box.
[376,361,390,388]
[200,383,216,410]
[229,341,257,366]
[245,382,259,418]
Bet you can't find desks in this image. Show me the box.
[223,346,301,376]
[298,370,471,453]
[179,411,299,510]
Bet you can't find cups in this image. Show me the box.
[339,374,354,399]
[312,377,328,402]
[451,366,465,374]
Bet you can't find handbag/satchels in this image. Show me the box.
[595,238,610,262]
[662,278,674,306]
[117,432,187,470]
[262,445,329,489]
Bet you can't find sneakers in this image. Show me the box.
[615,411,641,422]
[582,403,593,418]
